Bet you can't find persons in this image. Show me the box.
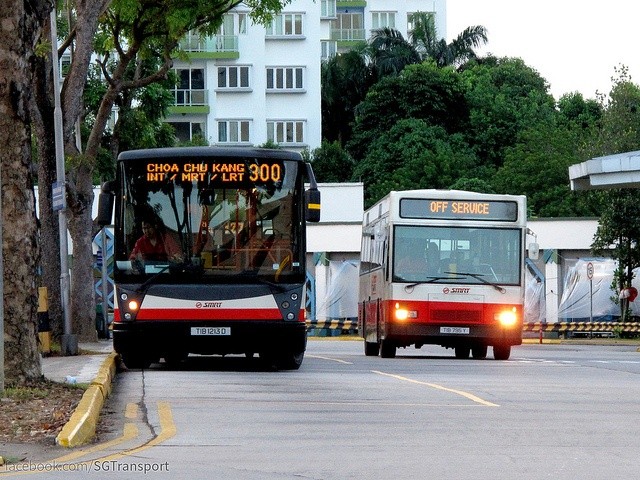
[128,216,183,265]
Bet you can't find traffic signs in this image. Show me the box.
[586,262,594,279]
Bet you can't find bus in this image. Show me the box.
[358,189,539,360]
[97,146,321,370]
